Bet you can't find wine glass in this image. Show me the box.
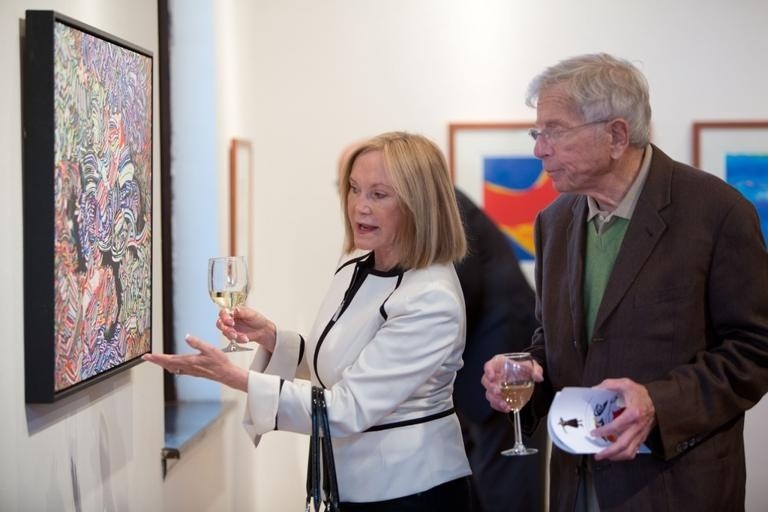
[497,351,539,458]
[207,256,253,356]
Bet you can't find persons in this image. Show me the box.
[479,53,767,512]
[335,138,544,512]
[140,130,475,512]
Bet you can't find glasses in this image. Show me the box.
[526,119,609,148]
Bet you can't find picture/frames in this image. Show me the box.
[21,7,154,404]
[446,120,564,291]
[228,138,256,308]
[690,118,768,246]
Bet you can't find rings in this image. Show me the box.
[175,367,182,374]
